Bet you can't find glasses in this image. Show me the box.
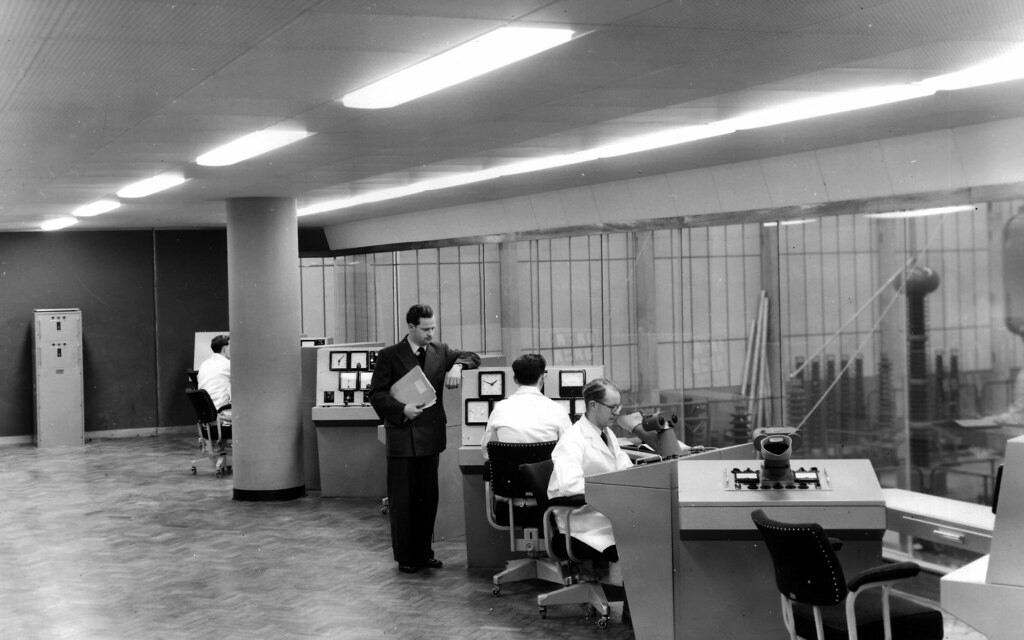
[542,370,548,376]
[594,398,622,415]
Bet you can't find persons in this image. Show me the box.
[482,353,572,528]
[546,379,634,629]
[198,335,232,426]
[369,304,481,571]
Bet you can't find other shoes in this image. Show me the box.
[399,562,419,574]
[421,557,442,567]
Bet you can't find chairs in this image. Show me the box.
[184,389,233,479]
[519,459,624,629]
[751,510,922,640]
[487,440,565,598]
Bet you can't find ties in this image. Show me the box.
[600,432,608,445]
[415,347,426,370]
